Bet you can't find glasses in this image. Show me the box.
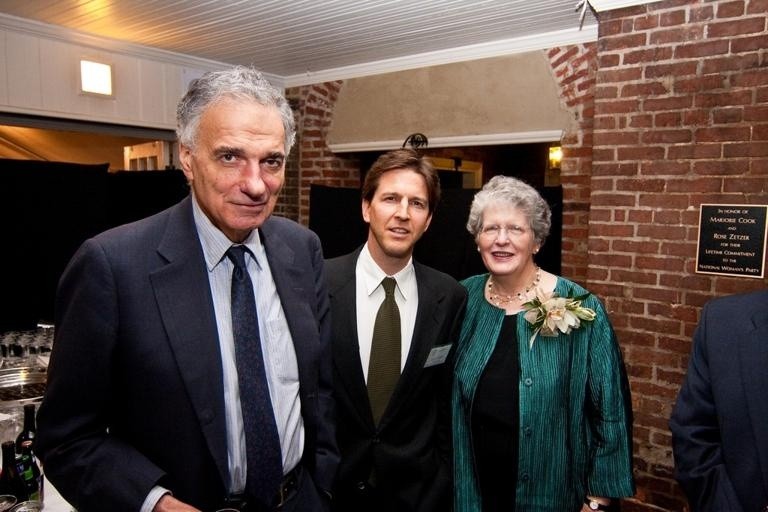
[480,224,534,238]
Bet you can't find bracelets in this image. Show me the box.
[583,497,608,511]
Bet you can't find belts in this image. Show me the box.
[219,466,304,511]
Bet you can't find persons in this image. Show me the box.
[29,59,342,511]
[444,173,636,512]
[317,150,471,510]
[667,284,768,511]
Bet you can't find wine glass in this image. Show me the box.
[0,327,55,369]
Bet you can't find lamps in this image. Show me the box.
[548,142,561,170]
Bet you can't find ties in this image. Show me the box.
[223,245,284,506]
[367,278,403,431]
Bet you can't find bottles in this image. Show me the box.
[0,403,46,504]
